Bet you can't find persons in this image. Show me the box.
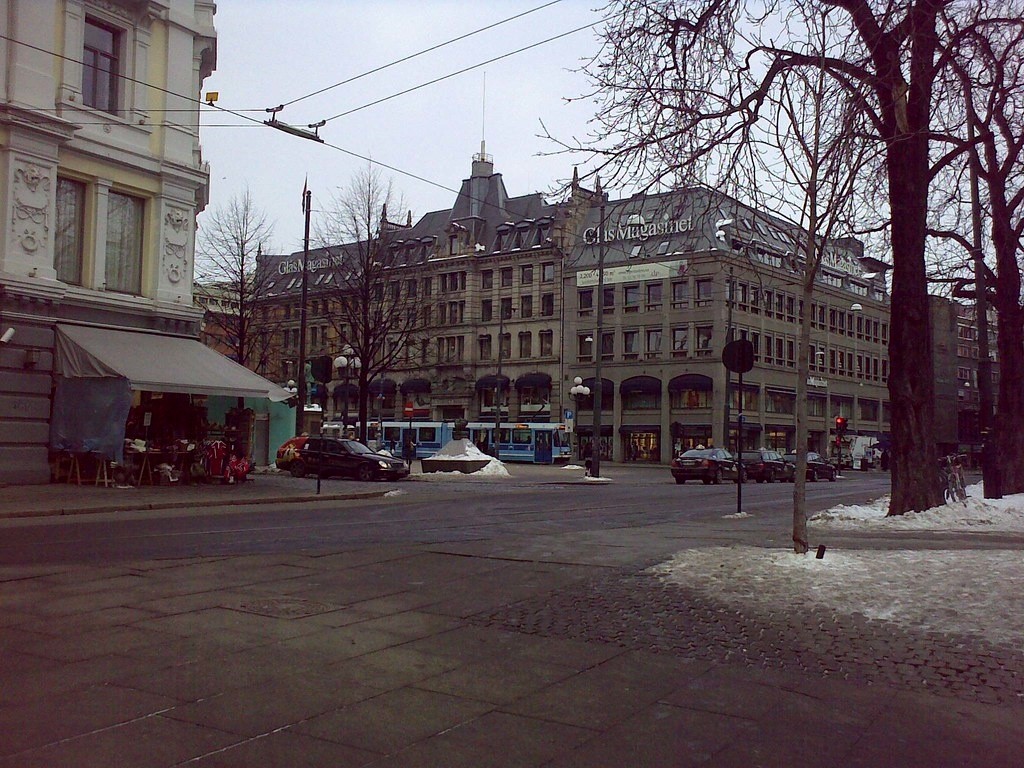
[582,442,594,475]
[880,448,890,471]
[845,445,852,468]
[632,442,638,461]
[303,356,315,408]
[405,437,418,466]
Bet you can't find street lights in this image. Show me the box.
[332,344,362,428]
[567,375,591,461]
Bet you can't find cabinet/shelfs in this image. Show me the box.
[224,412,255,460]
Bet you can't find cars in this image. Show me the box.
[671,444,747,485]
[783,452,837,483]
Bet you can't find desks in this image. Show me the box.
[90,449,190,488]
[64,448,93,486]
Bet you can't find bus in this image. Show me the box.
[355,418,572,465]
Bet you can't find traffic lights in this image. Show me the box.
[835,415,848,435]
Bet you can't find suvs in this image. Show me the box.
[275,432,410,482]
[735,449,797,483]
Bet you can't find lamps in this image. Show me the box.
[25,348,41,364]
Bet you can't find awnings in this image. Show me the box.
[765,424,796,432]
[514,372,551,392]
[475,375,509,393]
[574,425,612,433]
[830,428,857,435]
[304,384,327,397]
[729,422,764,431]
[619,425,660,433]
[332,384,359,400]
[576,378,614,397]
[55,322,294,402]
[668,374,713,391]
[400,378,431,395]
[859,430,882,436]
[619,376,661,398]
[369,379,397,396]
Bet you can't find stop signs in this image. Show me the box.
[403,402,414,417]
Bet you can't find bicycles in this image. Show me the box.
[937,453,967,505]
